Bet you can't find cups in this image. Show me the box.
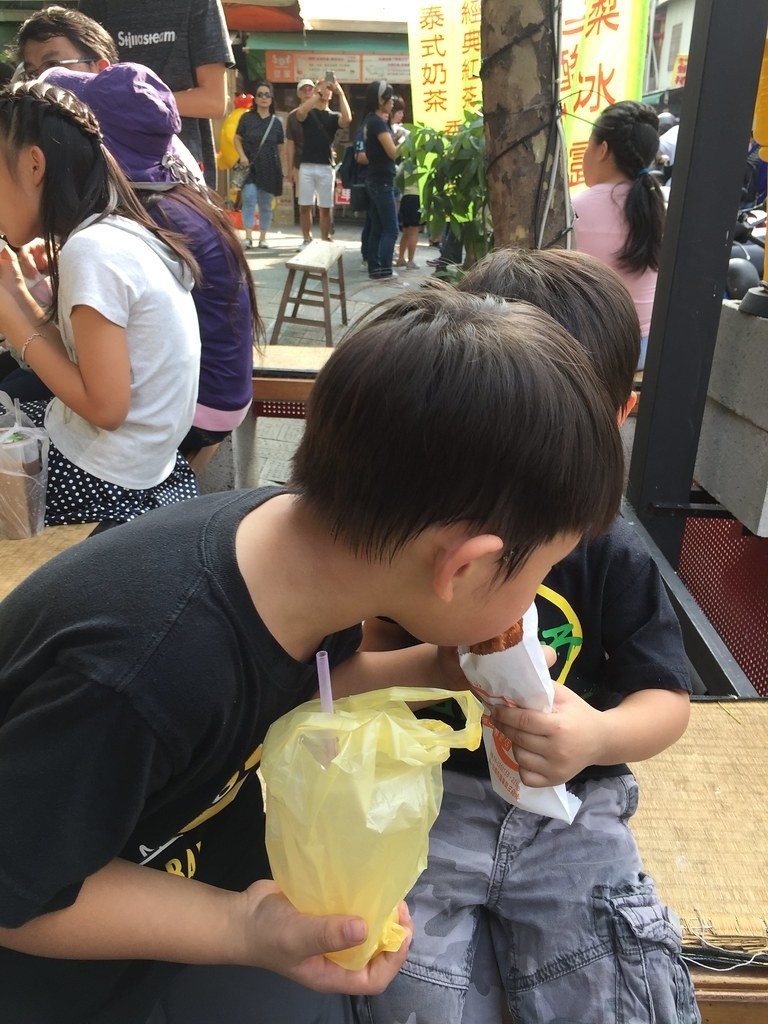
[0,425,46,540]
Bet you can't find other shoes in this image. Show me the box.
[397,263,421,270]
[359,264,368,271]
[371,272,399,281]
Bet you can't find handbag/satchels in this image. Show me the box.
[339,146,357,189]
[263,685,484,974]
[1,391,50,539]
[228,161,252,212]
[350,187,369,212]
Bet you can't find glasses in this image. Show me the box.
[256,92,273,98]
[18,57,98,83]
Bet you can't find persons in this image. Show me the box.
[337,247,703,1023]
[655,108,768,214]
[1,8,122,360]
[1,63,255,452]
[1,80,203,526]
[296,74,354,255]
[1,281,629,1023]
[566,100,675,373]
[353,105,390,269]
[386,95,411,264]
[365,81,403,281]
[285,80,337,239]
[395,158,424,265]
[427,221,488,278]
[218,92,254,210]
[73,1,237,196]
[232,83,287,250]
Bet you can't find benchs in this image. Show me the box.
[622,693,767,1024]
[0,440,222,606]
[252,344,645,423]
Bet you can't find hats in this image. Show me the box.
[34,61,184,189]
[297,78,314,89]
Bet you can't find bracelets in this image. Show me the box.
[317,92,323,97]
[19,334,47,364]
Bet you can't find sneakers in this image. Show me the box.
[296,238,311,251]
[259,242,268,248]
[426,259,439,266]
[243,238,252,248]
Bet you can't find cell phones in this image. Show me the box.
[326,72,333,83]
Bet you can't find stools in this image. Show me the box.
[269,238,348,347]
